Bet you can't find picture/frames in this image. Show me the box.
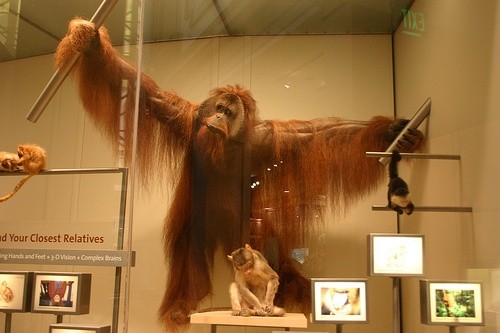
[367,233,425,278]
[0,271,33,312]
[49,324,111,333]
[31,271,91,315]
[311,278,369,324]
[420,279,485,326]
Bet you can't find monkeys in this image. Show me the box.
[226,244,285,318]
[0,144,46,202]
